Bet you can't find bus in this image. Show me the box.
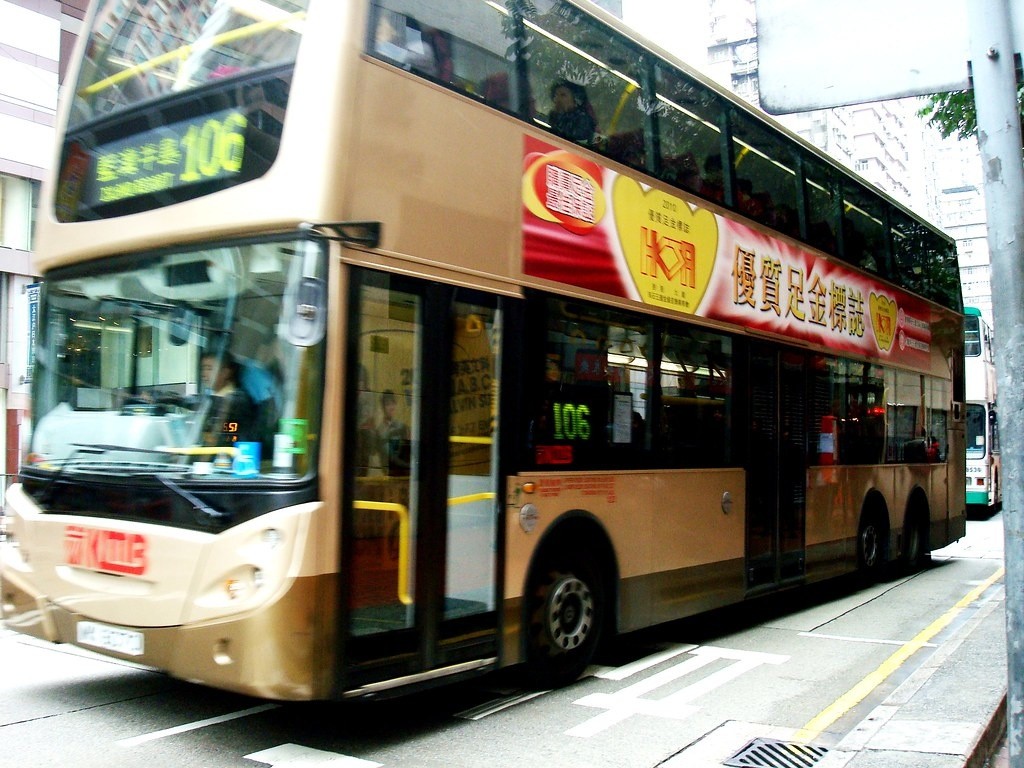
[0,0,968,708]
[963,306,1002,520]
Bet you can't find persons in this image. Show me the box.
[375,392,410,473]
[358,365,369,477]
[201,350,252,451]
[549,79,594,137]
